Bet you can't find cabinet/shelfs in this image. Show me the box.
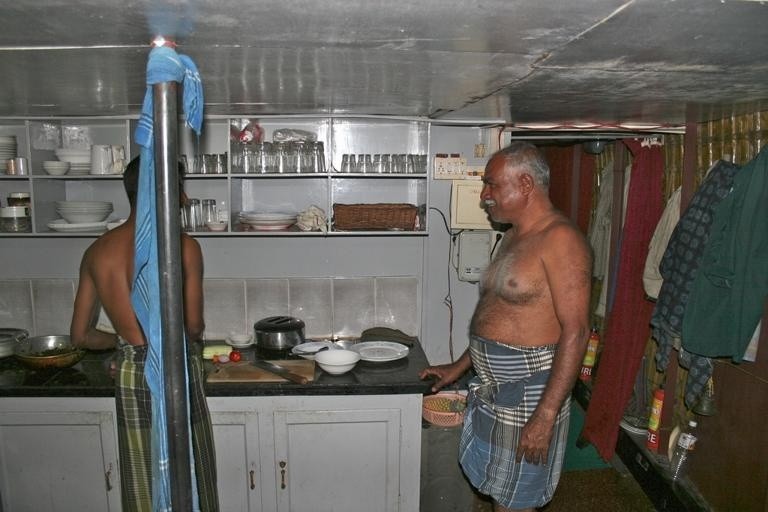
[0,111,431,237]
[269,393,421,512]
[204,396,269,512]
[1,395,123,512]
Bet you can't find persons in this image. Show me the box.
[66,150,222,512]
[419,140,597,510]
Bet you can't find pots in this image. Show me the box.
[13,334,89,370]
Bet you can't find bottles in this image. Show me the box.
[178,152,227,173]
[644,389,664,451]
[577,327,598,381]
[665,419,700,482]
[340,154,427,174]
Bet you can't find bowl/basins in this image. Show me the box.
[314,349,361,377]
[230,334,251,344]
[40,160,72,176]
[6,192,30,206]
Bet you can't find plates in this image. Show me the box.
[46,200,113,233]
[1,327,29,359]
[53,147,95,175]
[238,212,297,232]
[618,419,651,435]
[0,135,17,175]
[348,341,410,366]
[224,336,257,350]
[290,340,344,360]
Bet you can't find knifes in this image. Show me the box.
[248,358,308,386]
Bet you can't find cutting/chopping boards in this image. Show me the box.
[205,359,315,385]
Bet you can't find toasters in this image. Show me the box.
[253,313,306,352]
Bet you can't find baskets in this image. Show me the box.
[332,202,420,231]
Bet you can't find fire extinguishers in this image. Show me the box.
[579,328,600,381]
[646,383,665,450]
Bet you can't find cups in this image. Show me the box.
[176,199,216,231]
[0,205,30,233]
[229,141,327,173]
[90,144,126,176]
